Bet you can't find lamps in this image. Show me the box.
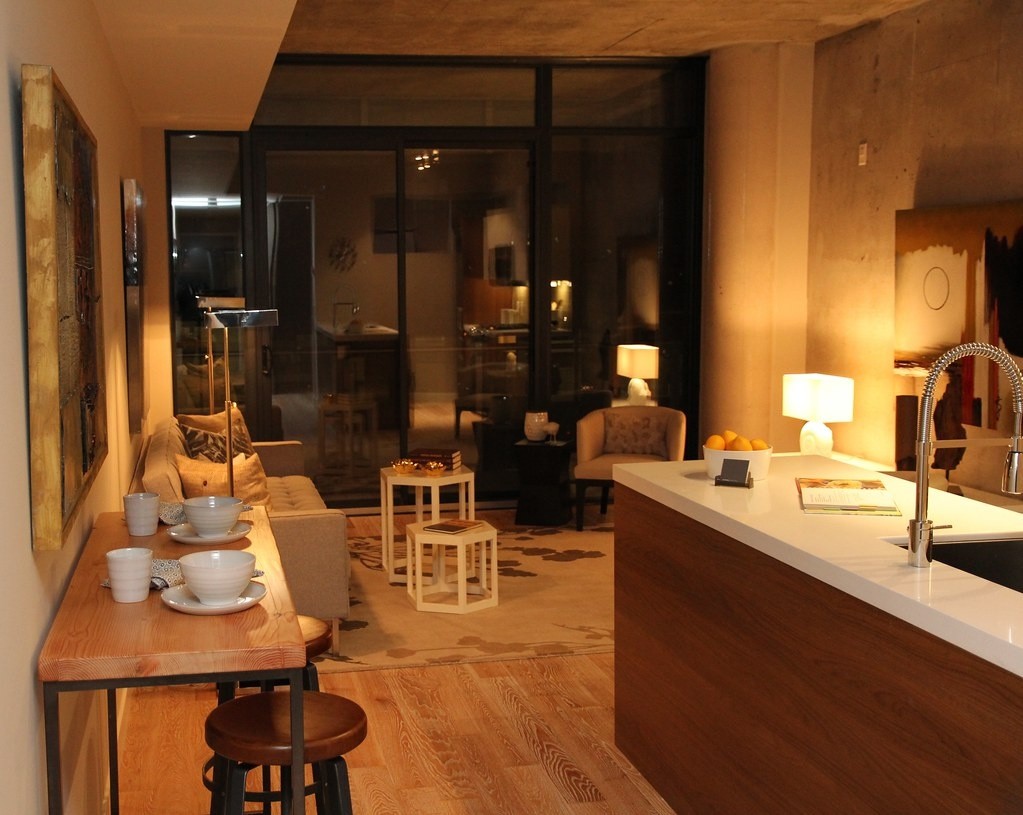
[204,310,279,512]
[196,296,245,416]
[616,344,660,406]
[782,373,855,455]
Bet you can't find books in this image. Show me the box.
[407,448,462,471]
[337,391,378,405]
[794,476,901,516]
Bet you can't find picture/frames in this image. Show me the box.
[20,62,109,552]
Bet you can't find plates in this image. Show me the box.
[166,520,252,545]
[162,581,267,615]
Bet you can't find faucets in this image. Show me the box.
[909,343,1023,567]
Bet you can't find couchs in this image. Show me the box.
[141,415,351,658]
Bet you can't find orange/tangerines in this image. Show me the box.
[705,430,768,451]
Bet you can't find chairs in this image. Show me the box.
[454,365,528,440]
[577,406,686,531]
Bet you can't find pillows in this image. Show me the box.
[174,403,275,513]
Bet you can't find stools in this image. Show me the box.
[216,614,334,815]
[204,690,367,815]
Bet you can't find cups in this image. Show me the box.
[106,548,153,603]
[524,411,549,442]
[123,493,160,536]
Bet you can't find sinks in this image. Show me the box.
[878,531,1022,594]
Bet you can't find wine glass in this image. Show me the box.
[547,423,559,443]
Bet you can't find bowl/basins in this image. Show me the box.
[390,458,419,474]
[179,550,256,605]
[182,496,243,537]
[420,461,447,476]
[703,444,773,481]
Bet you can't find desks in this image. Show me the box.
[318,402,381,472]
[406,518,499,614]
[380,465,475,584]
[39,505,306,815]
[514,432,577,524]
